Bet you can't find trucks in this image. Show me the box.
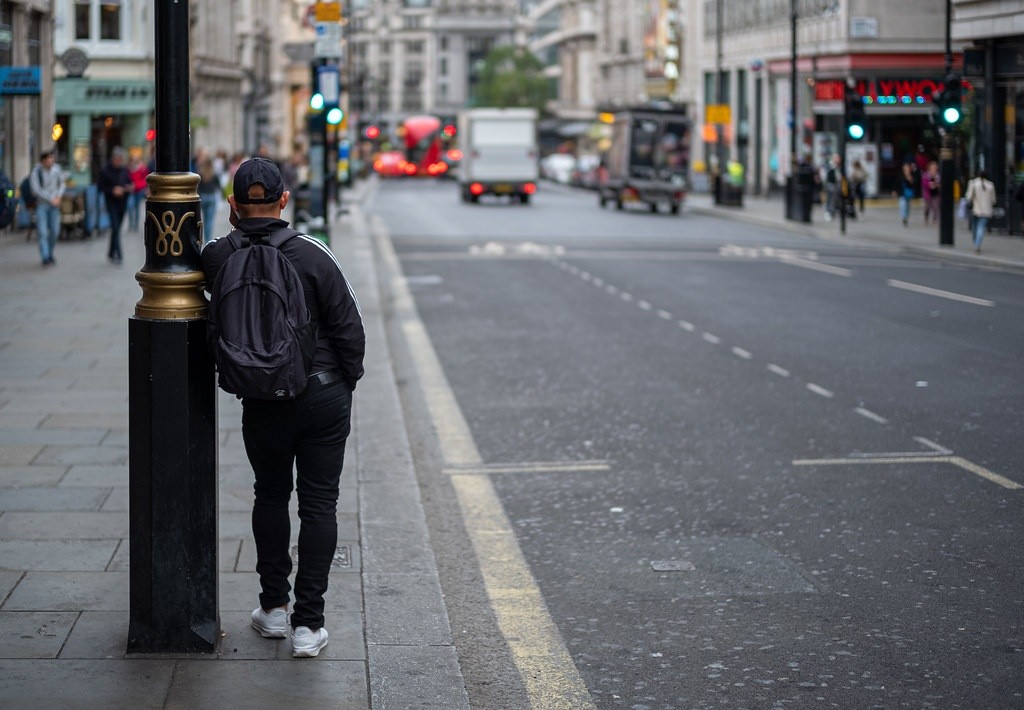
[592,99,695,214]
[456,106,541,203]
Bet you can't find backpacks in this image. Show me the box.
[206,228,323,401]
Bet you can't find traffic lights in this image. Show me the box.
[944,78,964,130]
[844,95,866,142]
[931,94,945,126]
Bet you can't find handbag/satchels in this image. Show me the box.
[903,187,913,200]
[20,167,43,207]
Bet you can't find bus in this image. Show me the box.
[538,117,603,187]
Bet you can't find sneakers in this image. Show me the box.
[250,605,294,638]
[290,625,328,657]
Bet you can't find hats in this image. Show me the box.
[233,157,283,204]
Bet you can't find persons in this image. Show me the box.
[890,154,941,230]
[800,153,869,222]
[95,145,134,266]
[127,141,311,245]
[29,152,65,267]
[201,156,366,657]
[964,168,997,252]
